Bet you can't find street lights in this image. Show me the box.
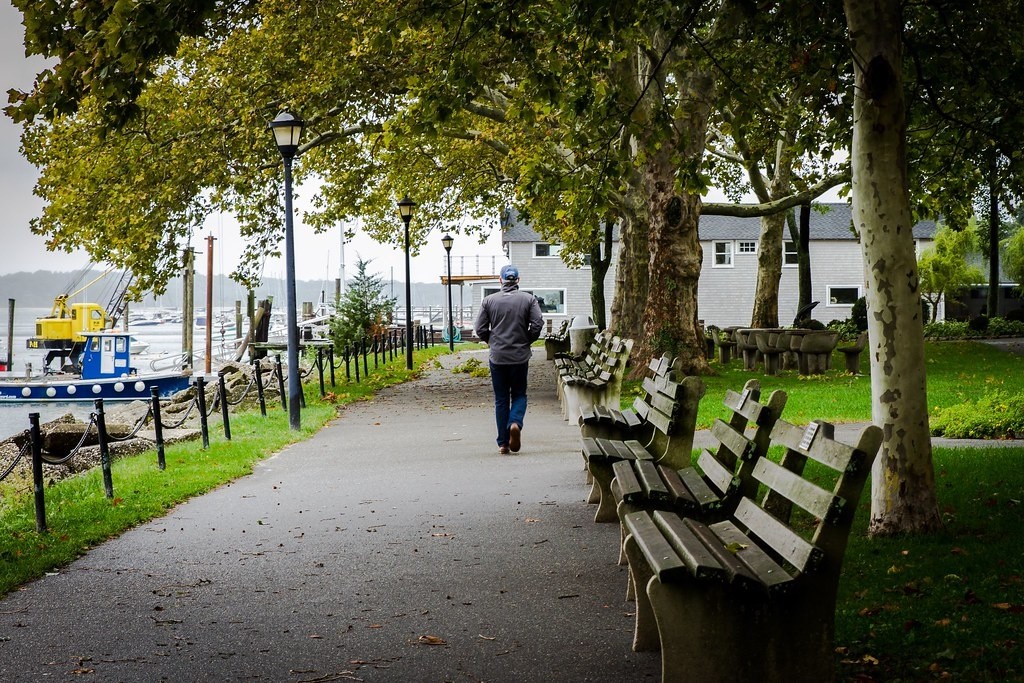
[397,194,417,371]
[442,233,454,352]
[269,106,307,431]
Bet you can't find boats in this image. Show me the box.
[0,331,191,406]
[104,299,306,360]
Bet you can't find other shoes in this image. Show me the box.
[510,424,521,452]
[499,447,509,454]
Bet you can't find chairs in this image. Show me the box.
[543,318,886,681]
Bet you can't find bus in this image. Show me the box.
[945,283,1023,322]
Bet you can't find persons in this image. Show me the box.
[472,262,546,456]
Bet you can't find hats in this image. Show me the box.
[500,265,518,281]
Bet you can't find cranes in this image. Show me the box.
[25,250,137,374]
